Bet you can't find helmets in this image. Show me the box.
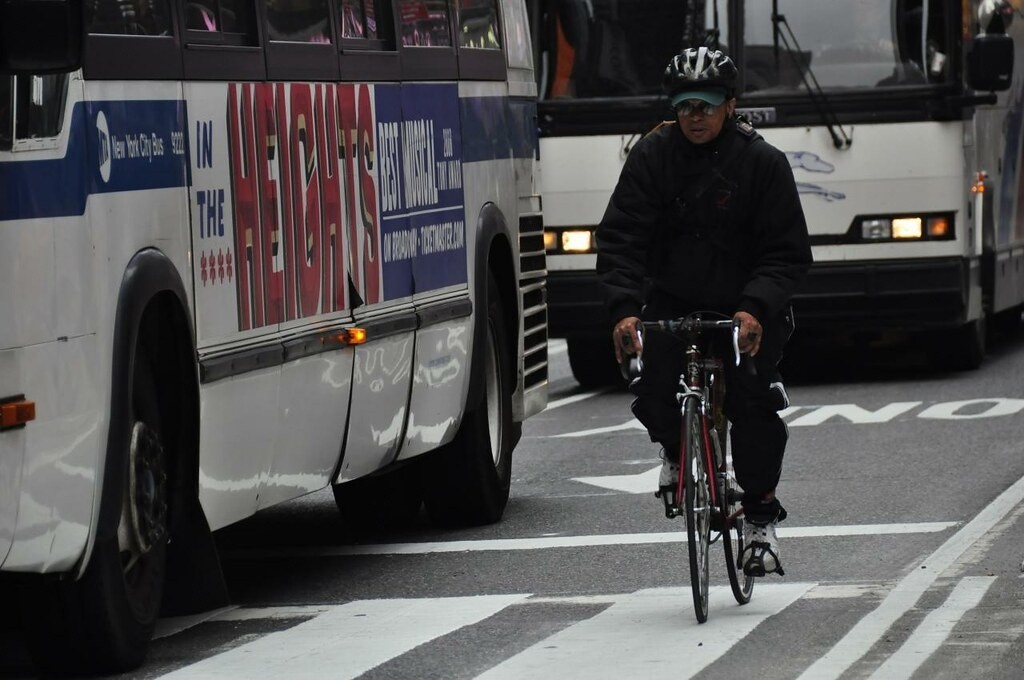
[661,47,740,97]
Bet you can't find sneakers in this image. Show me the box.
[657,443,686,508]
[740,517,781,576]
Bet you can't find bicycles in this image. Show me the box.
[620,311,785,626]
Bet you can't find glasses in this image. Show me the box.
[677,100,723,117]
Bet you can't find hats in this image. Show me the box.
[671,82,729,106]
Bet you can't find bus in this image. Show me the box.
[515,0,1024,394]
[0,0,556,680]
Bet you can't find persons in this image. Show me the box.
[596,45,814,577]
